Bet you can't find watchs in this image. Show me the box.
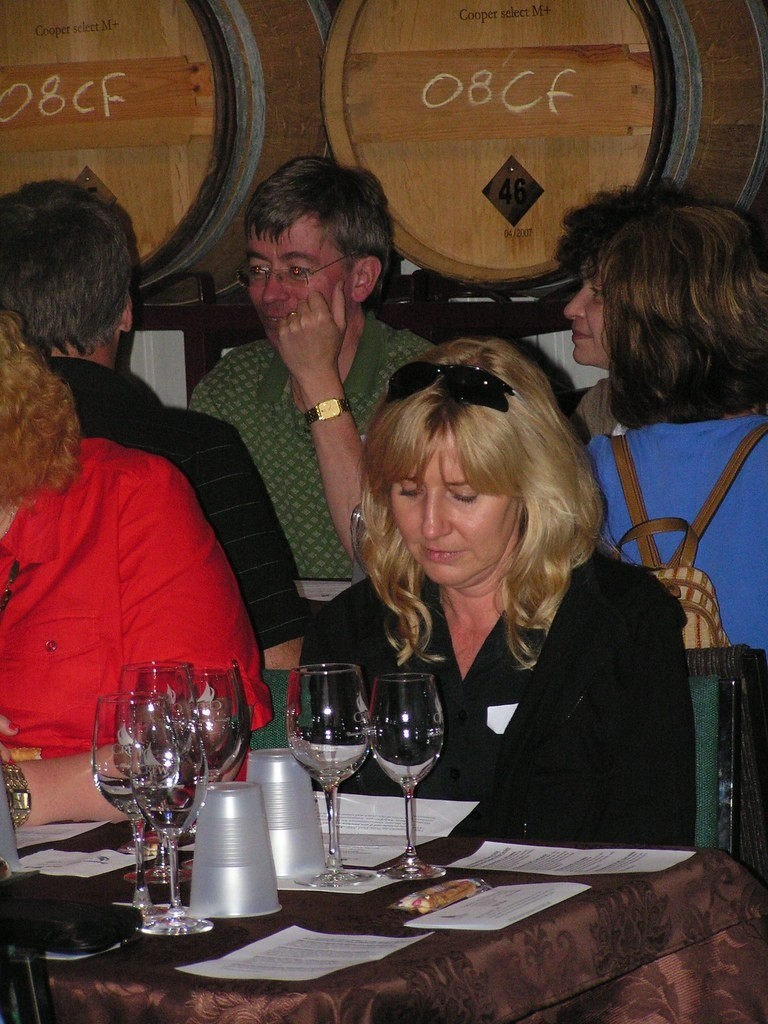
[1,764,31,830]
[304,398,351,425]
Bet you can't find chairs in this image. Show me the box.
[244,667,741,874]
[120,300,616,418]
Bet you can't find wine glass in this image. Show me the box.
[285,663,376,888]
[367,671,447,880]
[171,659,250,870]
[91,661,214,936]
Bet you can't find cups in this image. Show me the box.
[246,748,328,879]
[186,782,282,920]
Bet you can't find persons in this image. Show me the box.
[190,153,436,581]
[0,177,309,839]
[298,337,698,864]
[552,184,768,654]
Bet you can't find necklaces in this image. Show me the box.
[0,506,15,539]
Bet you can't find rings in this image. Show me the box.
[287,311,297,319]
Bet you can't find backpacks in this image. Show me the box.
[610,421,768,650]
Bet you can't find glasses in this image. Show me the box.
[236,252,355,289]
[385,361,527,412]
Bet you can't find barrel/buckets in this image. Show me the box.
[321,0,766,294]
[0,0,331,309]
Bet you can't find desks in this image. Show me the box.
[0,817,768,1024]
